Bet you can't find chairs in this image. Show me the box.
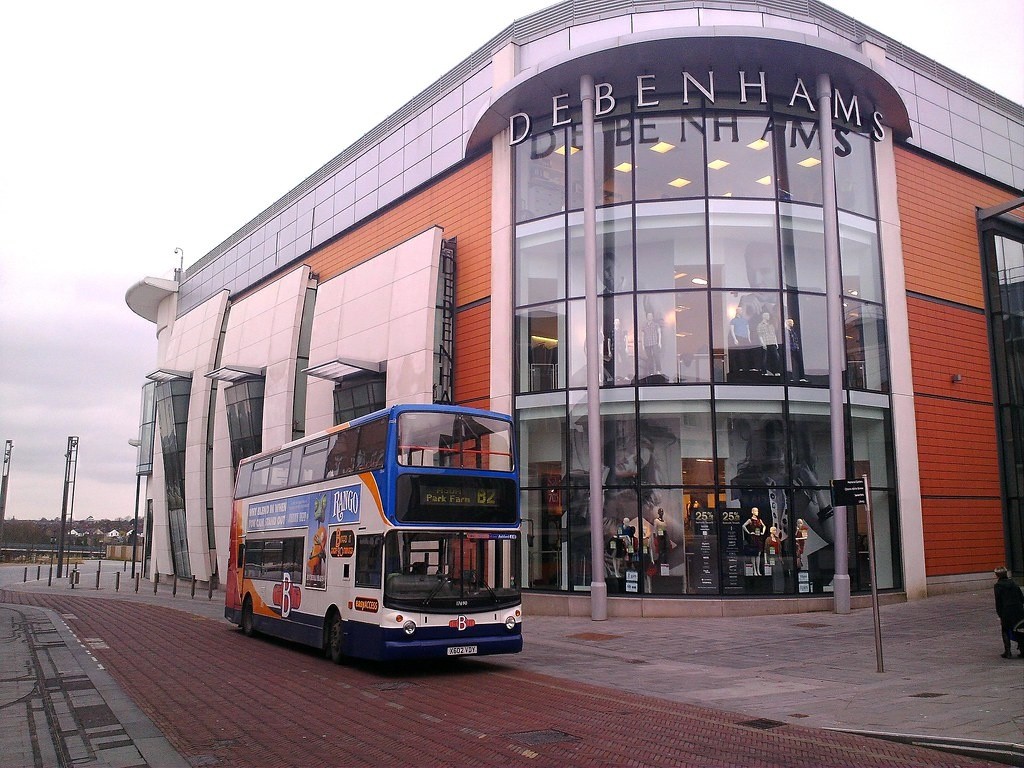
[237,444,483,496]
[241,530,428,597]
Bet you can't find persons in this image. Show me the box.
[565,419,683,590]
[764,527,785,593]
[730,307,758,372]
[653,508,669,567]
[607,317,630,381]
[757,313,780,376]
[994,566,1024,658]
[728,241,791,375]
[606,517,635,578]
[638,526,655,593]
[730,420,835,590]
[642,312,662,375]
[786,319,807,382]
[795,519,808,570]
[743,508,766,576]
[571,251,666,386]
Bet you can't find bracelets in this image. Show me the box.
[817,505,834,521]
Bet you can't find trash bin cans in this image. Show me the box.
[68,568,80,584]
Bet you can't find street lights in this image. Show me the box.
[174,247,184,272]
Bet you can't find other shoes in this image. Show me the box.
[1001,652,1012,658]
[1017,653,1024,658]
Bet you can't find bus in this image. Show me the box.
[224,404,534,664]
